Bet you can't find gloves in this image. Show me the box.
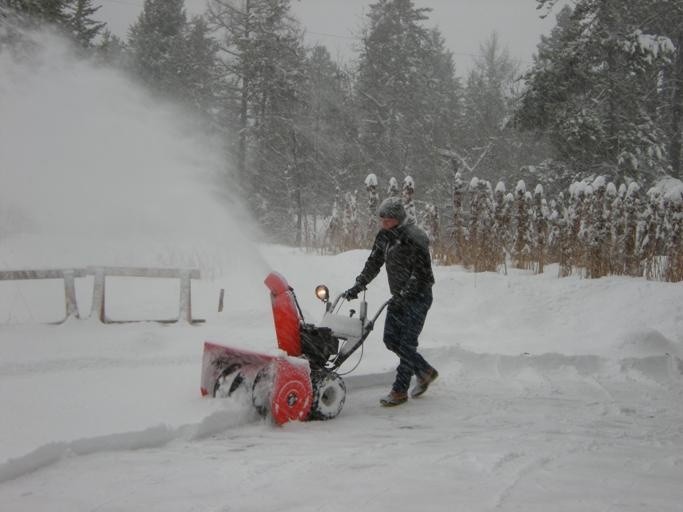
[342,281,367,302]
[387,291,410,312]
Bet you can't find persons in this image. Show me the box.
[342,199,438,407]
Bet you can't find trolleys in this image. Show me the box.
[202,273,399,425]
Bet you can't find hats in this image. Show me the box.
[379,197,407,220]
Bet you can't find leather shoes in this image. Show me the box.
[379,390,408,408]
[410,365,439,399]
